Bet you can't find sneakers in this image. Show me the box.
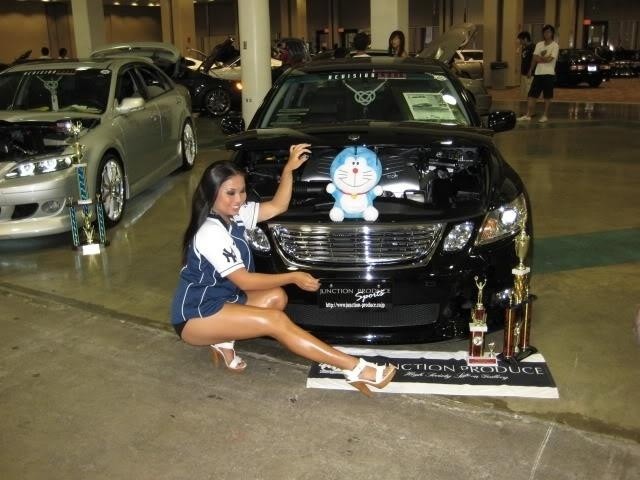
[516,111,549,122]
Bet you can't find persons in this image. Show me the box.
[170,142,396,399]
[57,48,70,59]
[276,42,294,65]
[388,30,407,57]
[517,31,537,117]
[352,32,372,58]
[38,46,53,60]
[516,24,559,122]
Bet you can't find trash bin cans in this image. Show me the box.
[490,62,508,90]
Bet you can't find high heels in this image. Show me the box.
[208,340,248,373]
[342,357,397,398]
